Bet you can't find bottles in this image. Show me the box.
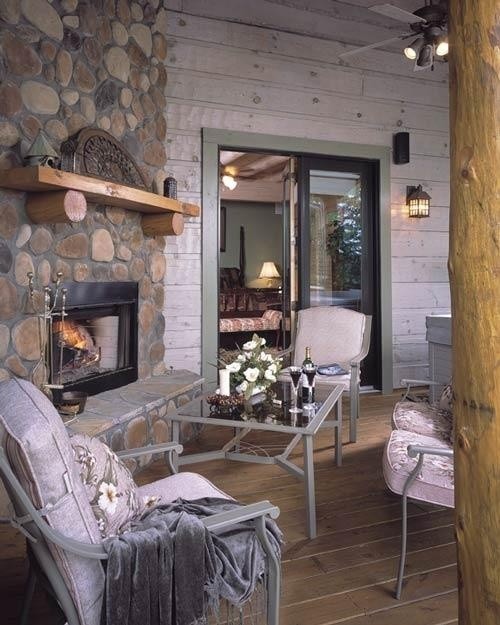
[302,346,317,407]
[164,176,177,199]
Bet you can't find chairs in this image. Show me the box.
[275,306,374,444]
[0,376,286,624]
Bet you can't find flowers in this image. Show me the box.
[217,330,285,383]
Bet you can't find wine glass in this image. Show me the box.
[287,366,319,413]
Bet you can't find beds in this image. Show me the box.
[221,267,282,318]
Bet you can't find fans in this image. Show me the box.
[330,0,450,59]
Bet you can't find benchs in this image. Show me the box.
[384,360,456,598]
[219,310,283,350]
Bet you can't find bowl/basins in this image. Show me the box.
[23,154,59,168]
[62,391,88,414]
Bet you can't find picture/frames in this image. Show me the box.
[220,206,228,251]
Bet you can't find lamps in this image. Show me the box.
[220,169,237,192]
[404,181,431,220]
[259,260,279,288]
[404,24,450,72]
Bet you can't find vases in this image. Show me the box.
[235,379,267,395]
